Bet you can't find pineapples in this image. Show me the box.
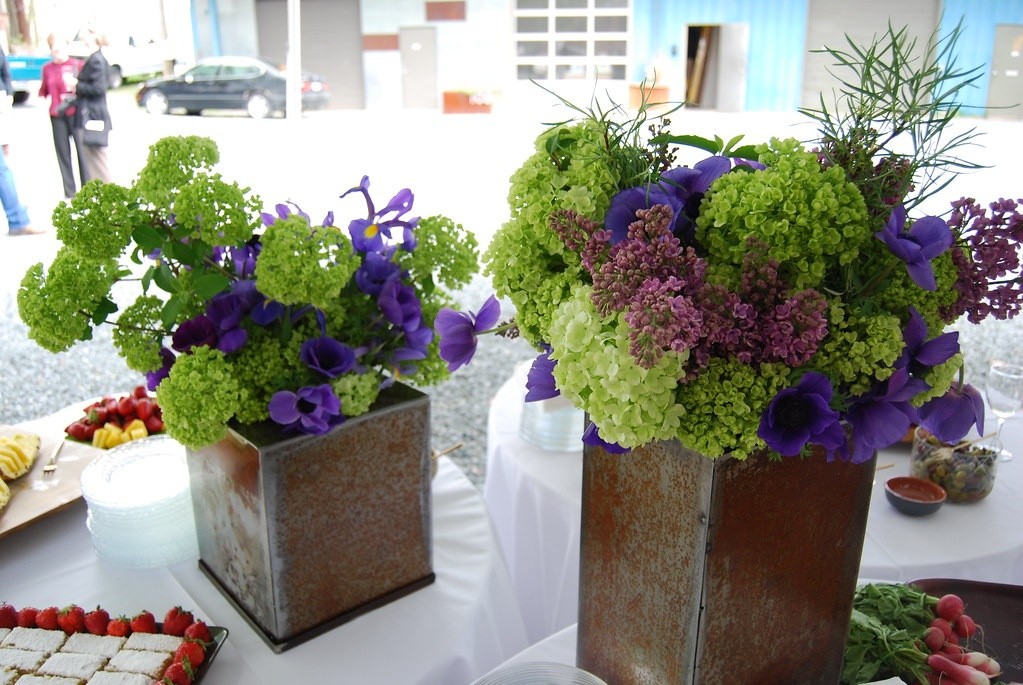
[92,419,148,449]
[0,433,41,510]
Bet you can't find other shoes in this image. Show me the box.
[8,225,42,235]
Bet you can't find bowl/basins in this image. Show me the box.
[911,426,1003,502]
[886,477,949,517]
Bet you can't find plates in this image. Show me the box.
[79,434,200,570]
[474,662,609,684]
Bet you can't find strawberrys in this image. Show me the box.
[0,601,157,637]
[66,387,164,439]
[153,605,212,685]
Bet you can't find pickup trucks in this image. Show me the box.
[0,51,54,106]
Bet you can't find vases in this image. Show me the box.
[184,371,441,658]
[629,85,669,110]
[443,89,491,115]
[576,411,878,685]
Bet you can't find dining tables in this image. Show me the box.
[0,380,530,685]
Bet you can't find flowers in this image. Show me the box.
[433,5,1023,465]
[18,134,479,452]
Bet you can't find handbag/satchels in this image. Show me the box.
[81,116,108,147]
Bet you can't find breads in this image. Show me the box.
[0,626,184,685]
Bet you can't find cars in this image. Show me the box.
[61,26,184,89]
[136,56,331,118]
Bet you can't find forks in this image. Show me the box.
[43,440,64,476]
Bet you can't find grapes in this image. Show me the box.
[917,436,997,503]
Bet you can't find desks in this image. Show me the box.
[457,579,1023,685]
[488,344,1023,641]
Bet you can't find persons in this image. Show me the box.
[38,24,113,200]
[0,44,44,236]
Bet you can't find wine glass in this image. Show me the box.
[975,363,1023,463]
